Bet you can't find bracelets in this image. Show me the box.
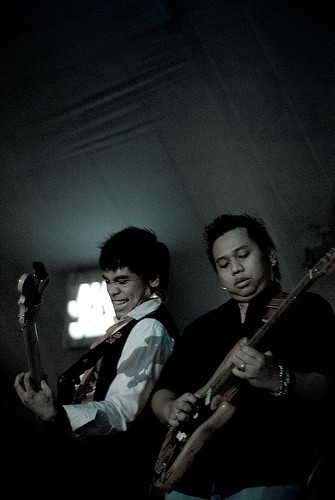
[271,364,289,399]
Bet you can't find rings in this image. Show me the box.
[238,361,246,371]
[174,408,182,419]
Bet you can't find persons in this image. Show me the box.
[152,212,334,499]
[14,227,177,500]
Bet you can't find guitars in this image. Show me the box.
[16,261,53,393]
[153,247,335,492]
